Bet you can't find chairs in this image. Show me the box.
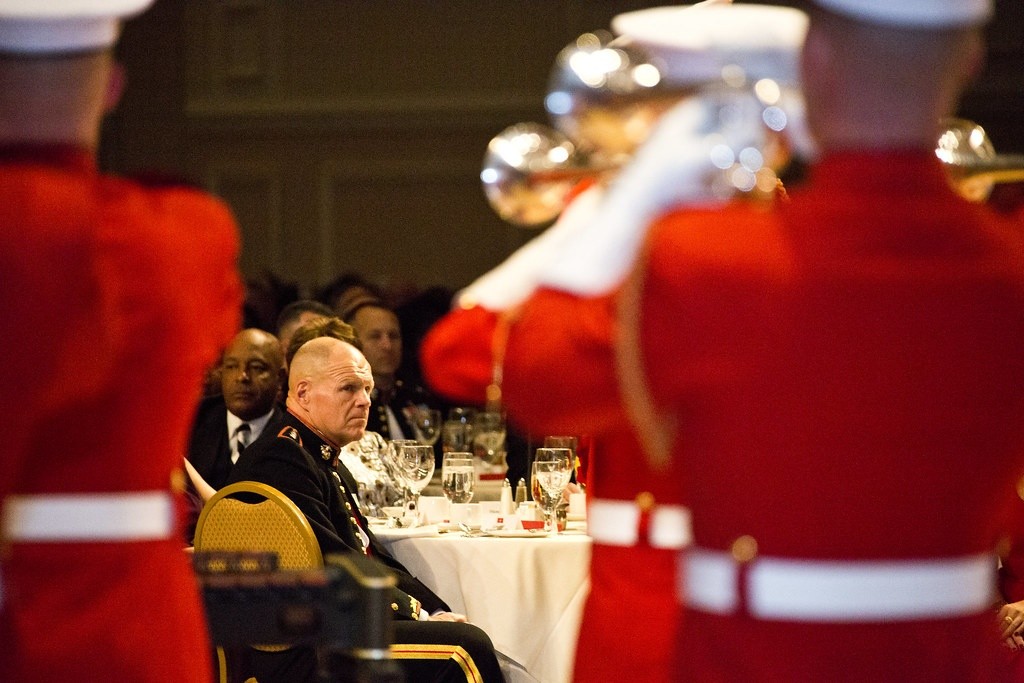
[195,482,326,683]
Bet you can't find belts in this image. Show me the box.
[583,499,692,550]
[681,553,994,621]
[4,492,173,538]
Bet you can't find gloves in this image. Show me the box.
[602,87,765,218]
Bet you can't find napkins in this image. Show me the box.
[369,525,441,544]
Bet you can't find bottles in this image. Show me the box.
[501,478,514,518]
[515,478,527,508]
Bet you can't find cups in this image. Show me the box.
[449,504,481,527]
[443,453,474,504]
[569,494,586,514]
[420,497,447,522]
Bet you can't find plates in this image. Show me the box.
[567,513,587,521]
[493,530,549,538]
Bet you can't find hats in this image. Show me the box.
[611,2,811,87]
[822,1,993,29]
[0,2,155,55]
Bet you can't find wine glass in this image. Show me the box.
[388,440,435,526]
[414,408,506,465]
[531,436,576,538]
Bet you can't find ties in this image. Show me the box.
[231,424,250,465]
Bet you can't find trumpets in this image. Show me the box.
[935,117,1024,203]
[478,30,792,231]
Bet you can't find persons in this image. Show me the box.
[184,273,578,681]
[420,0,1024,681]
[0,0,243,683]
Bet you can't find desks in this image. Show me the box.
[366,502,592,683]
[422,477,504,502]
